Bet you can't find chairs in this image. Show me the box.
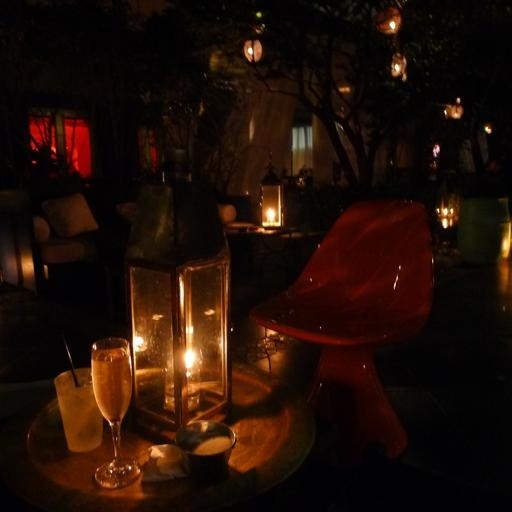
[249,198,435,460]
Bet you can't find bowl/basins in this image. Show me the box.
[175,421,237,471]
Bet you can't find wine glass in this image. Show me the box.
[91,337,141,489]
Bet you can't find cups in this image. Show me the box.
[54,368,103,452]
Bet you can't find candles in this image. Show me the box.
[44,264,49,280]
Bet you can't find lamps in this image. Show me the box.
[242,38,264,63]
[291,104,315,175]
[260,168,285,228]
[128,182,233,449]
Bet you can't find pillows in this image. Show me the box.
[40,193,99,238]
